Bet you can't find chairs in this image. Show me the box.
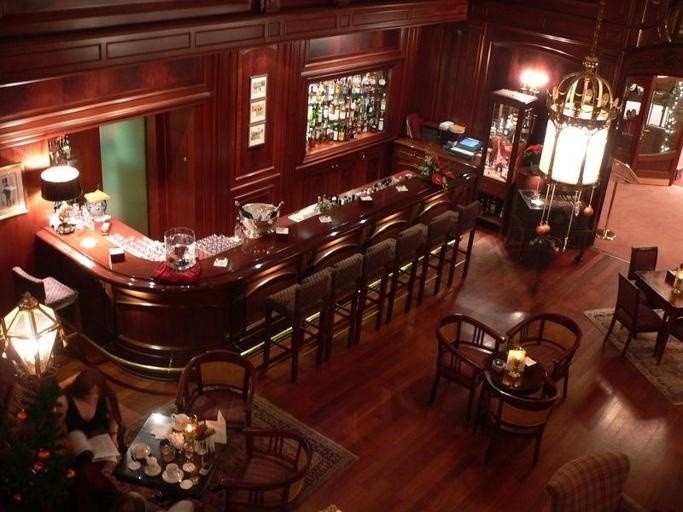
[220,427,313,510]
[500,175,598,267]
[263,273,331,381]
[604,272,667,355]
[304,200,479,361]
[627,246,660,281]
[507,314,583,397]
[12,266,82,333]
[177,350,256,432]
[480,370,559,466]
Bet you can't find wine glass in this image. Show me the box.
[182,441,195,472]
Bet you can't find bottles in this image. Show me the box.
[483,103,533,177]
[267,201,285,221]
[314,175,403,216]
[505,357,524,390]
[235,200,255,220]
[184,413,198,433]
[672,263,683,296]
[478,192,504,222]
[305,68,388,151]
[102,232,242,264]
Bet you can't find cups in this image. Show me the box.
[133,442,180,480]
[405,173,412,180]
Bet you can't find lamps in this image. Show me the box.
[528,55,624,255]
[1,291,68,398]
[40,162,82,234]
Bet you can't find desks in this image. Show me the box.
[507,189,596,267]
[112,411,227,508]
[635,269,683,356]
[483,352,546,395]
[42,170,463,370]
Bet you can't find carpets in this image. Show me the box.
[584,306,682,406]
[105,377,359,510]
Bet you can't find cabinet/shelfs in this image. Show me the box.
[389,88,538,228]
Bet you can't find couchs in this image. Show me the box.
[549,453,644,512]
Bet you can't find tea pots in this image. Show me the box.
[170,413,193,432]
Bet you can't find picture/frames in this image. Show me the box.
[0,162,28,223]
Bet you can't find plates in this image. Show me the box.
[128,443,194,490]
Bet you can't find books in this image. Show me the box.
[65,429,121,464]
